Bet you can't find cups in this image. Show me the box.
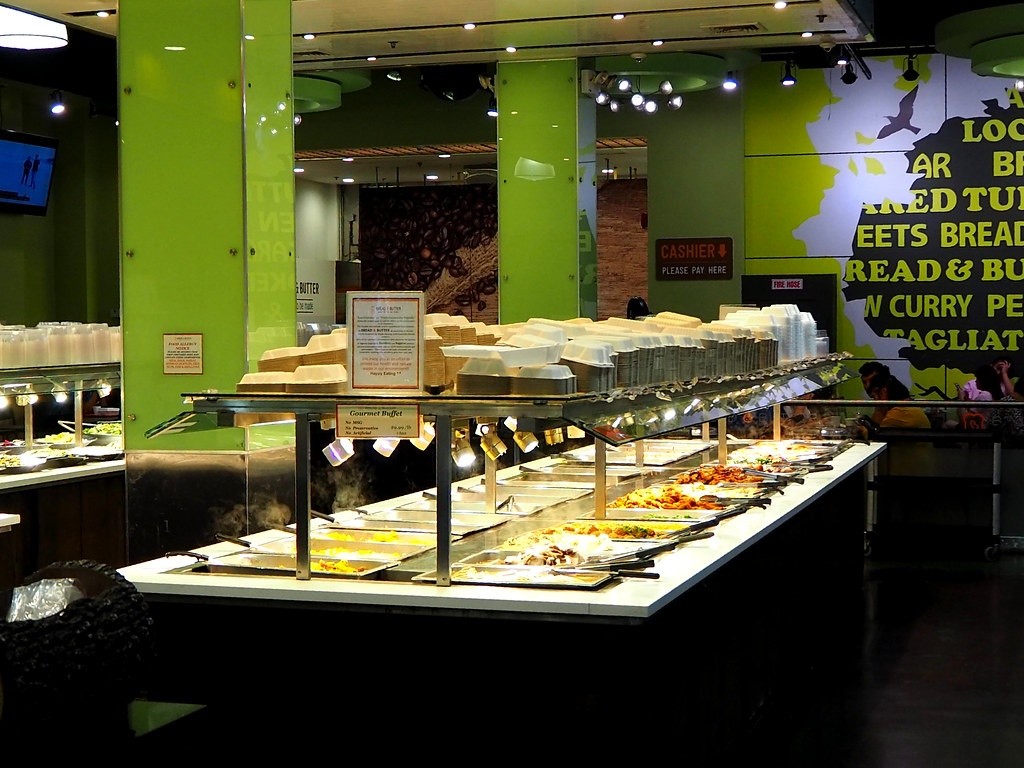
[832,416,840,428]
[93,406,101,415]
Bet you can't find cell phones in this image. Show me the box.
[955,383,961,389]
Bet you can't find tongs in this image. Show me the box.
[58,420,97,432]
[497,495,517,512]
[550,437,869,579]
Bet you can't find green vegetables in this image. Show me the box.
[82,422,122,436]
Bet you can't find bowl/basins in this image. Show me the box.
[237,304,829,399]
[100,408,119,415]
[0,433,123,474]
[846,418,856,426]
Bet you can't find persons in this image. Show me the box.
[955,360,1024,433]
[856,362,935,476]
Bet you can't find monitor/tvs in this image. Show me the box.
[0,129,59,216]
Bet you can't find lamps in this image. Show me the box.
[478,73,498,118]
[386,68,403,81]
[48,89,65,114]
[580,43,925,118]
